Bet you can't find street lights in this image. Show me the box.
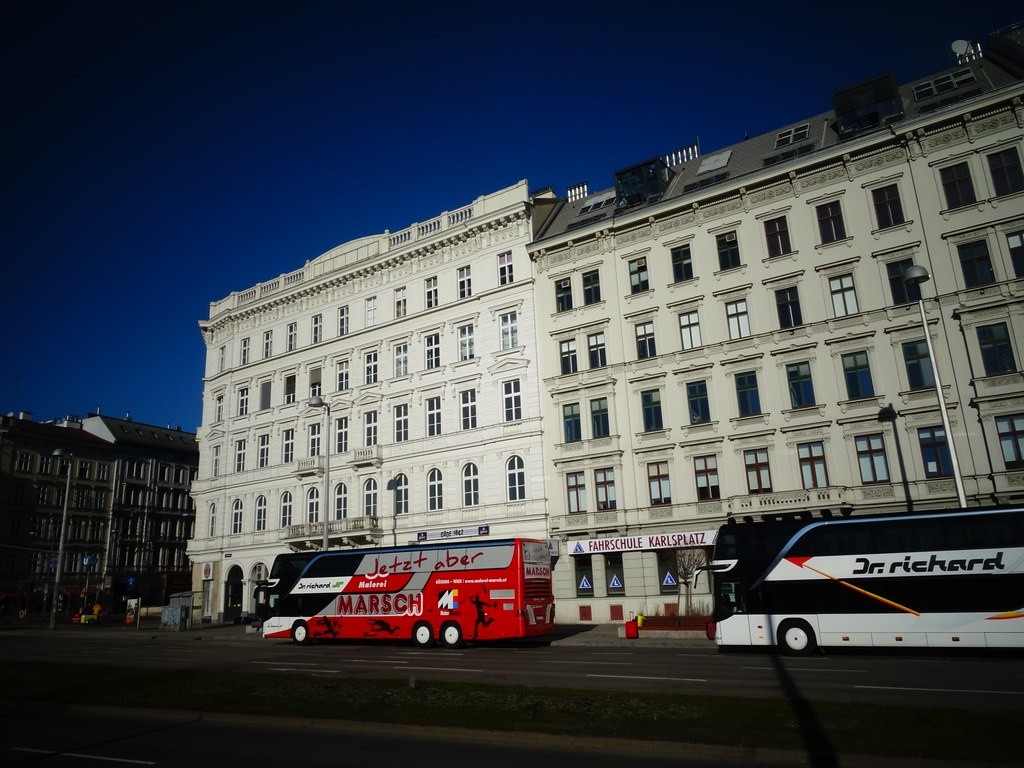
[307,397,330,551]
[905,264,970,511]
[50,449,74,630]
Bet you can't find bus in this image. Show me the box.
[691,504,1024,656]
[252,537,557,650]
